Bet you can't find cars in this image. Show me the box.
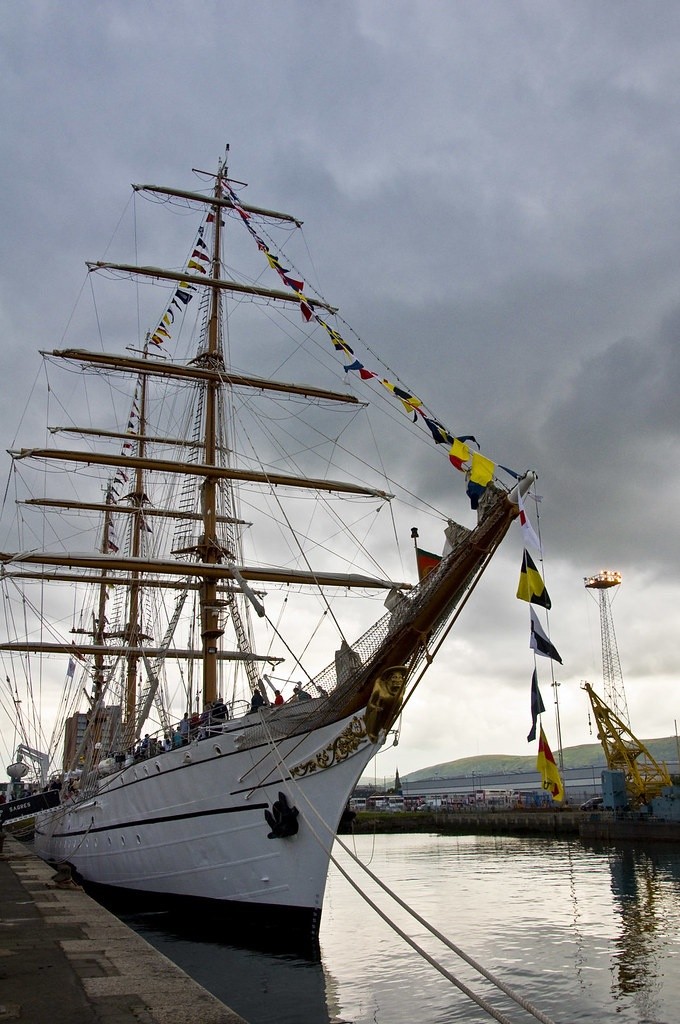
[579,798,604,811]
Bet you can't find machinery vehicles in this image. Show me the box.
[577,682,680,822]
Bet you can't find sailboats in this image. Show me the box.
[1,140,539,961]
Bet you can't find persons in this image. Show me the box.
[115,687,311,763]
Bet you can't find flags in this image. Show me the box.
[417,547,443,589]
[149,203,224,349]
[516,545,553,609]
[537,722,566,803]
[466,450,500,507]
[516,489,544,554]
[380,375,424,423]
[526,667,547,743]
[62,641,86,678]
[224,178,375,385]
[529,603,565,665]
[448,435,480,475]
[87,562,117,720]
[101,372,144,556]
[425,414,484,448]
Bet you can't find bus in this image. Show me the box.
[347,794,404,811]
